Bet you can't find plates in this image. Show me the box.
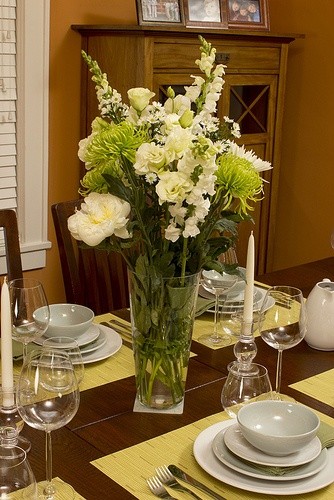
[23,322,122,365]
[192,420,334,496]
[198,284,275,315]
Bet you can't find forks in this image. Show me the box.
[146,475,179,500]
[154,465,203,500]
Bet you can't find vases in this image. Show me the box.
[127,260,200,410]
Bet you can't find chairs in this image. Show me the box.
[0,192,162,326]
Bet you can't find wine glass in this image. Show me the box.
[7,278,50,398]
[259,286,307,403]
[197,247,239,346]
[16,349,80,500]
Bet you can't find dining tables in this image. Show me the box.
[1,256,333,499]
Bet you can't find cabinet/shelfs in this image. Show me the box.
[67,23,295,281]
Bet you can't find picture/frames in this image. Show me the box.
[133,0,271,31]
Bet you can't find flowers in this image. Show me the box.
[65,33,275,405]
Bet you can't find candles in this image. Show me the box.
[241,230,254,337]
[1,276,16,407]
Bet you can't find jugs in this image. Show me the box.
[299,279,334,351]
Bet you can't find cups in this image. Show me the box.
[0,444,38,500]
[38,337,85,394]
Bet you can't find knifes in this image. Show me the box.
[168,465,227,500]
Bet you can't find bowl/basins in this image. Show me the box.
[32,303,94,343]
[237,400,320,457]
[201,267,247,297]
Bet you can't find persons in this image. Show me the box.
[228,0,260,22]
[200,0,220,22]
[141,0,180,22]
